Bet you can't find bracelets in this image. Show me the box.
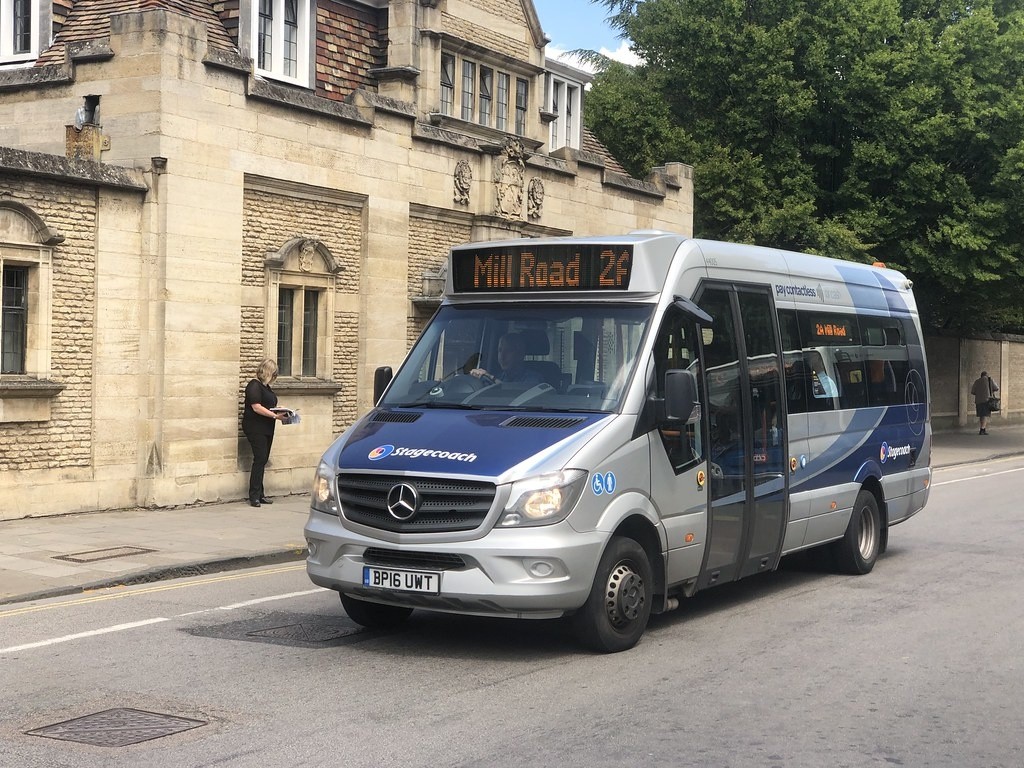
[273,415,277,420]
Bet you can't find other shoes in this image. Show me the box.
[260,498,273,504]
[251,500,260,506]
[979,431,989,435]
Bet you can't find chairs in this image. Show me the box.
[517,331,560,393]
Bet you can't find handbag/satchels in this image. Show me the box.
[989,397,1001,411]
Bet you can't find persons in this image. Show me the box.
[242,358,291,507]
[470,333,537,391]
[971,371,999,435]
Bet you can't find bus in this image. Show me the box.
[303,229,932,655]
